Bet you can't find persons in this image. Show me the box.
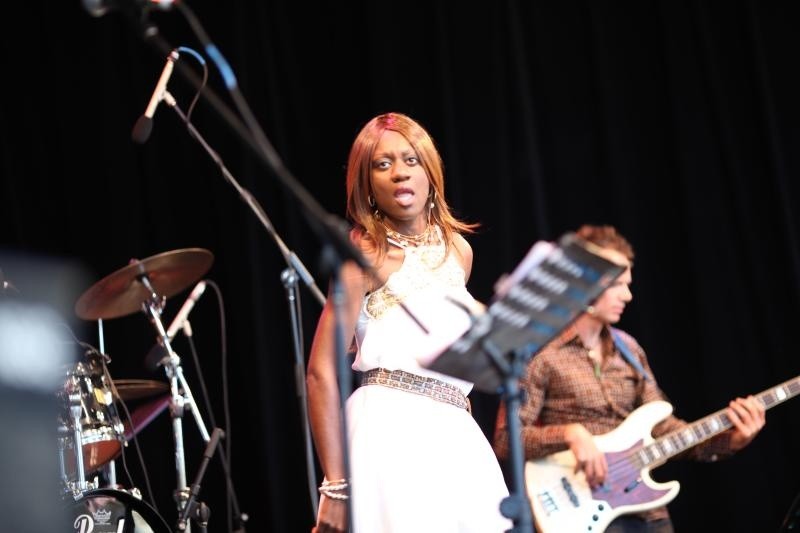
[493,226,765,533]
[305,112,521,533]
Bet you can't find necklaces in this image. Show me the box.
[379,219,436,249]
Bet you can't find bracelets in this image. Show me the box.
[318,479,350,500]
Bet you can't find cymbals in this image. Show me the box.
[74,247,214,321]
[110,378,170,398]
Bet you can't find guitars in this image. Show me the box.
[524,375,800,533]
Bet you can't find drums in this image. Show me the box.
[56,489,173,533]
[39,359,126,483]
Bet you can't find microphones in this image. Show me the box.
[131,51,178,144]
[84,0,179,18]
[143,282,207,373]
